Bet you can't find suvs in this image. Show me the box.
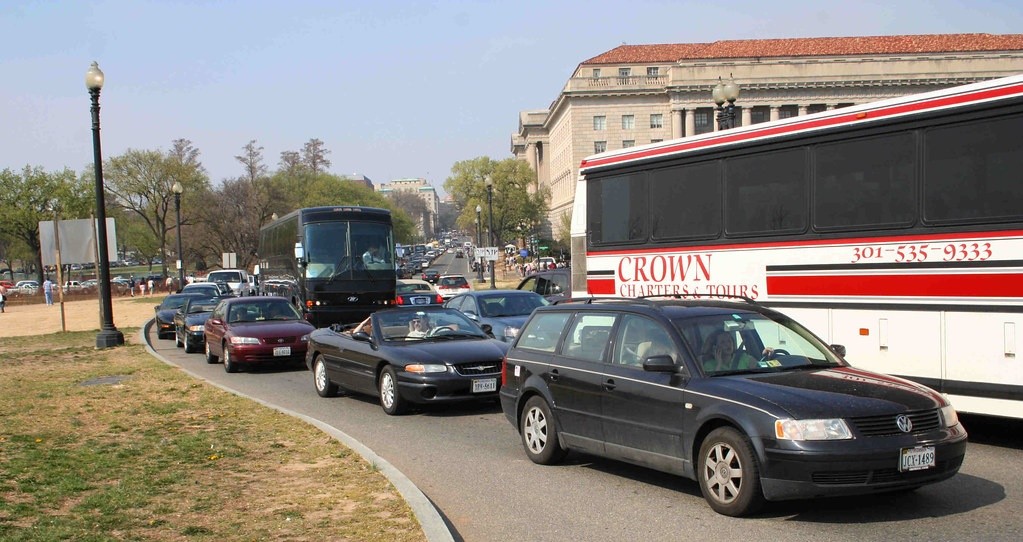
[524,257,556,271]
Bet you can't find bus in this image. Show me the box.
[257,206,397,329]
[571,73,1023,424]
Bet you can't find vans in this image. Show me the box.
[207,270,250,297]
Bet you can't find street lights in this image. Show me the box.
[476,205,485,283]
[84,61,125,348]
[485,175,496,290]
[713,73,739,131]
[474,218,480,278]
[172,182,184,293]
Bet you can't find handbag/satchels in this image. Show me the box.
[2,295,7,301]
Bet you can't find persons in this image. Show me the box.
[268,303,280,320]
[234,307,247,321]
[362,242,385,265]
[166,275,174,295]
[127,275,155,297]
[702,329,774,374]
[43,277,53,306]
[353,316,393,342]
[0,282,6,313]
[520,260,566,276]
[405,313,460,341]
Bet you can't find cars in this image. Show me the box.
[305,306,509,416]
[499,293,968,518]
[396,229,472,278]
[398,271,571,343]
[0,277,130,295]
[155,270,317,372]
[421,270,440,283]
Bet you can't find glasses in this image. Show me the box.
[413,320,421,323]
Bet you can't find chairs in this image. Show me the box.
[635,341,650,363]
[700,332,714,364]
[409,318,428,332]
[485,302,503,314]
